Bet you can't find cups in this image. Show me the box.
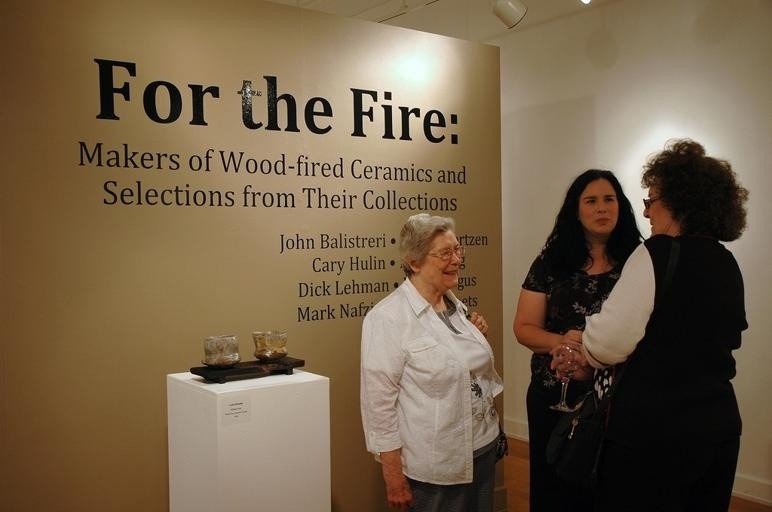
[251,328,289,361]
[200,334,241,369]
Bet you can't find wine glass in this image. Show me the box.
[548,343,582,414]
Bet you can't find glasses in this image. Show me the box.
[642,197,658,209]
[428,246,469,260]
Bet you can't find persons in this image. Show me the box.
[549,137,749,511]
[514,169,647,511]
[359,213,504,510]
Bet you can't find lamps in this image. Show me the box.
[487,0,528,29]
